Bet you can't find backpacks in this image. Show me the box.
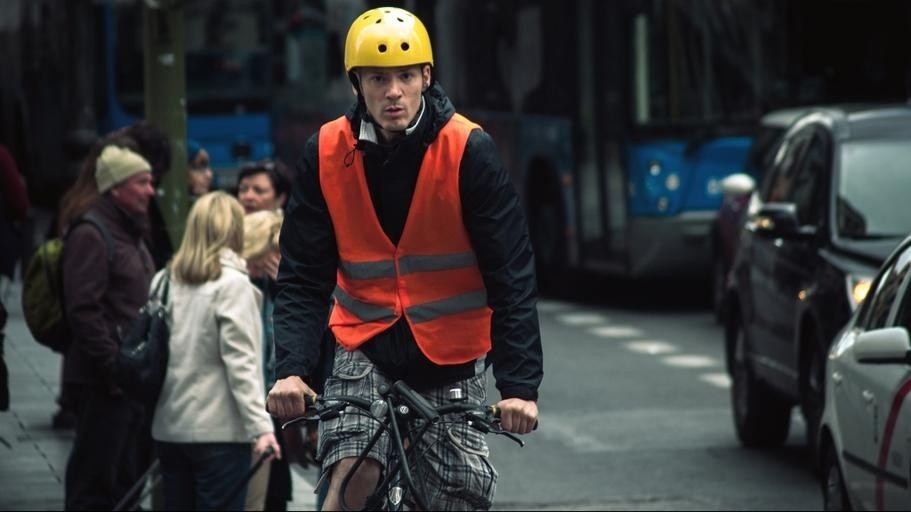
[22,213,117,354]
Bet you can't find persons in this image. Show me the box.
[265,4,543,512]
[2,120,319,512]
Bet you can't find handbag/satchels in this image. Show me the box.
[115,266,171,401]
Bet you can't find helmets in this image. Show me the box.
[342,7,435,92]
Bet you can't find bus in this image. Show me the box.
[0,0,268,191]
[268,0,749,300]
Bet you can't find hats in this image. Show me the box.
[95,144,151,195]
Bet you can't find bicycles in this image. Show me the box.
[266,392,539,511]
